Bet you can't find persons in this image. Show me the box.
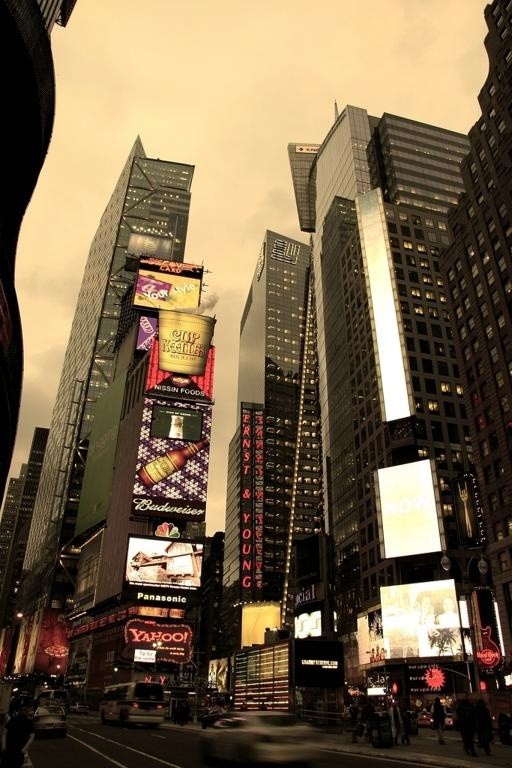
[348,697,411,746]
[454,697,480,759]
[472,700,496,757]
[1,701,36,768]
[431,701,447,746]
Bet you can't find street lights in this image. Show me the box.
[440,544,488,700]
[1,611,24,677]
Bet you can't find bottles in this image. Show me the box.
[168,416,182,438]
[137,436,210,489]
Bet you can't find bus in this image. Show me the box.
[99,680,164,728]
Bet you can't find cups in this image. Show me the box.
[158,310,217,376]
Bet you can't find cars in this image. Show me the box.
[3,673,89,739]
[406,710,458,731]
[196,711,325,767]
